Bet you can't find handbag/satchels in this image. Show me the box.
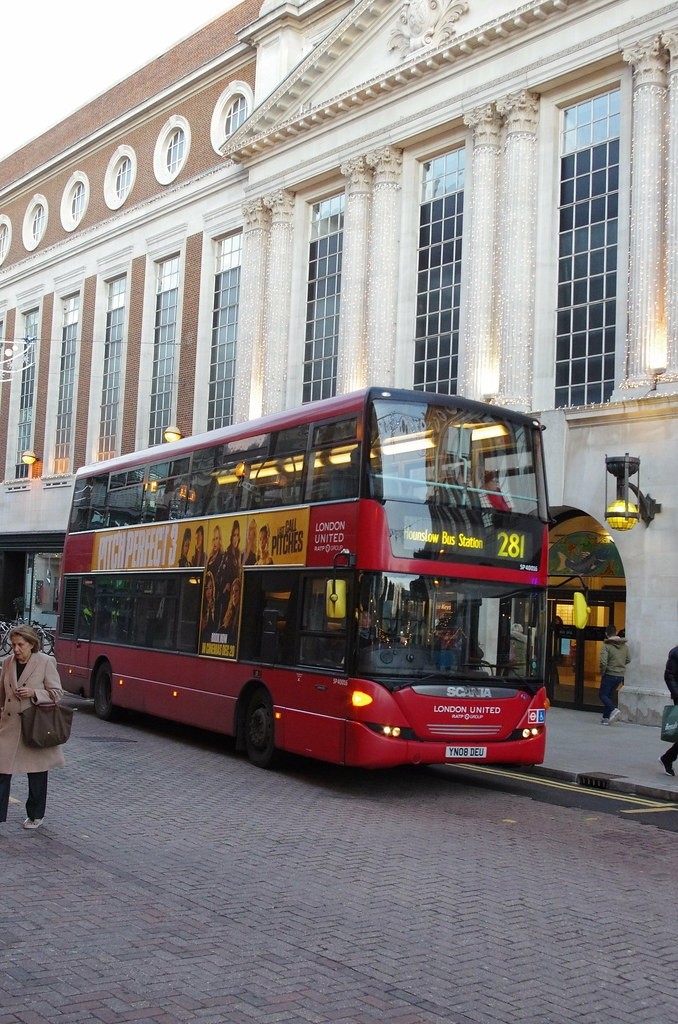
[18,690,73,747]
[660,704,678,742]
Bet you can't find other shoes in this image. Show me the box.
[22,817,44,828]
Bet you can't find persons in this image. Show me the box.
[177,517,274,646]
[599,625,632,726]
[657,645,678,776]
[0,625,64,829]
[358,609,408,647]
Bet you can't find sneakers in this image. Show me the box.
[659,756,675,776]
[609,708,621,723]
[600,718,609,724]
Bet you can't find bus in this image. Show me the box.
[53,385,592,777]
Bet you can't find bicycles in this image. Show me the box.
[0,616,56,657]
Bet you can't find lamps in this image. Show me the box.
[164,425,184,443]
[605,453,662,534]
[22,450,39,465]
